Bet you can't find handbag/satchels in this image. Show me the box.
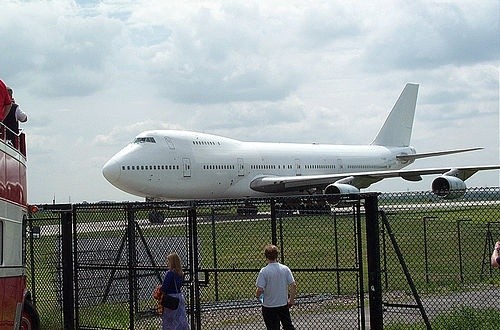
[161,294,179,310]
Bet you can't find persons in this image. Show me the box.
[157,253,190,330]
[490,241,500,267]
[0,79,27,150]
[255,244,297,330]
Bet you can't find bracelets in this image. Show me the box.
[493,248,500,252]
[255,296,259,298]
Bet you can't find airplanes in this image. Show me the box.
[101,82,500,226]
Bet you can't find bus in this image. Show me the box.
[0,121,42,330]
